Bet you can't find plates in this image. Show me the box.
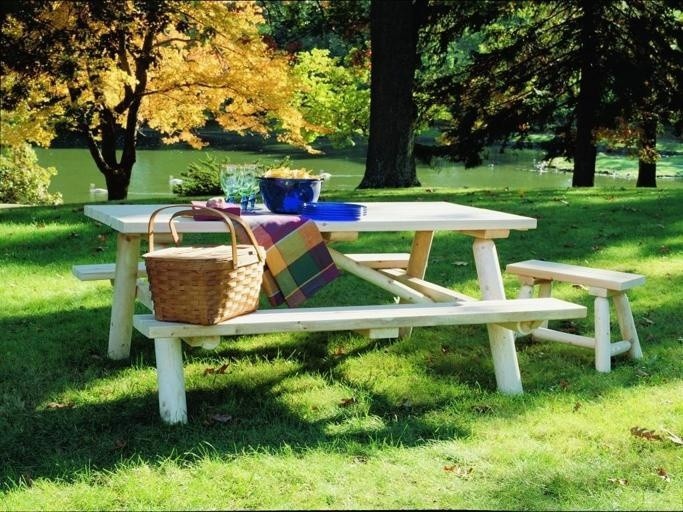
[302,201,367,222]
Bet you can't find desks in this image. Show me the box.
[83,200,537,426]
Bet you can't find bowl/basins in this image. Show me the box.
[257,177,324,214]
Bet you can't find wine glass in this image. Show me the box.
[219,164,264,215]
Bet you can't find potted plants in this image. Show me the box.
[219,163,323,215]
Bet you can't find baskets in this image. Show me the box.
[141,202,267,328]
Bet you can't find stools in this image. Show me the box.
[505,258,647,372]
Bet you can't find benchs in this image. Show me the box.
[73,252,410,360]
[132,297,589,426]
